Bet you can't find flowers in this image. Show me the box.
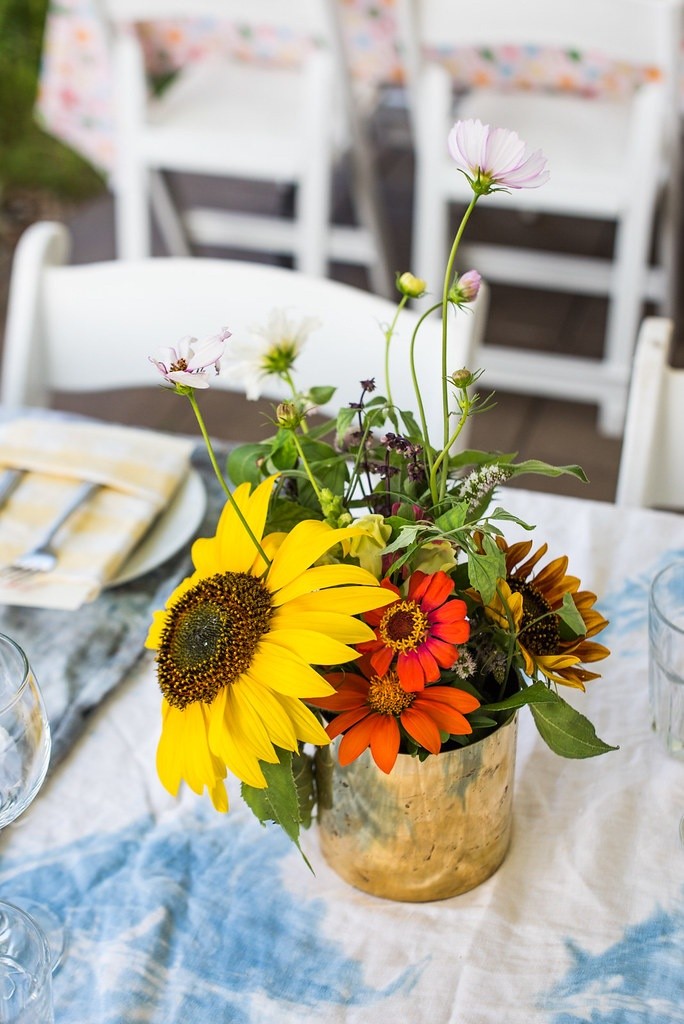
[142,117,620,874]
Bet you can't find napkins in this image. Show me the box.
[0,414,199,611]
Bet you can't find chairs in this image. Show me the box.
[398,0,683,434]
[98,0,391,301]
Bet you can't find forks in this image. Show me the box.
[1,477,97,585]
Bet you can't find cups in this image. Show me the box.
[648,559,683,762]
[0,901,55,1024]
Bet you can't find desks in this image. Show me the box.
[34,0,684,194]
[0,405,684,1024]
[1,222,490,460]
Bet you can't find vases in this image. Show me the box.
[317,713,516,904]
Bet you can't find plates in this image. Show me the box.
[104,465,208,588]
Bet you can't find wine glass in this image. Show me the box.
[0,633,69,975]
